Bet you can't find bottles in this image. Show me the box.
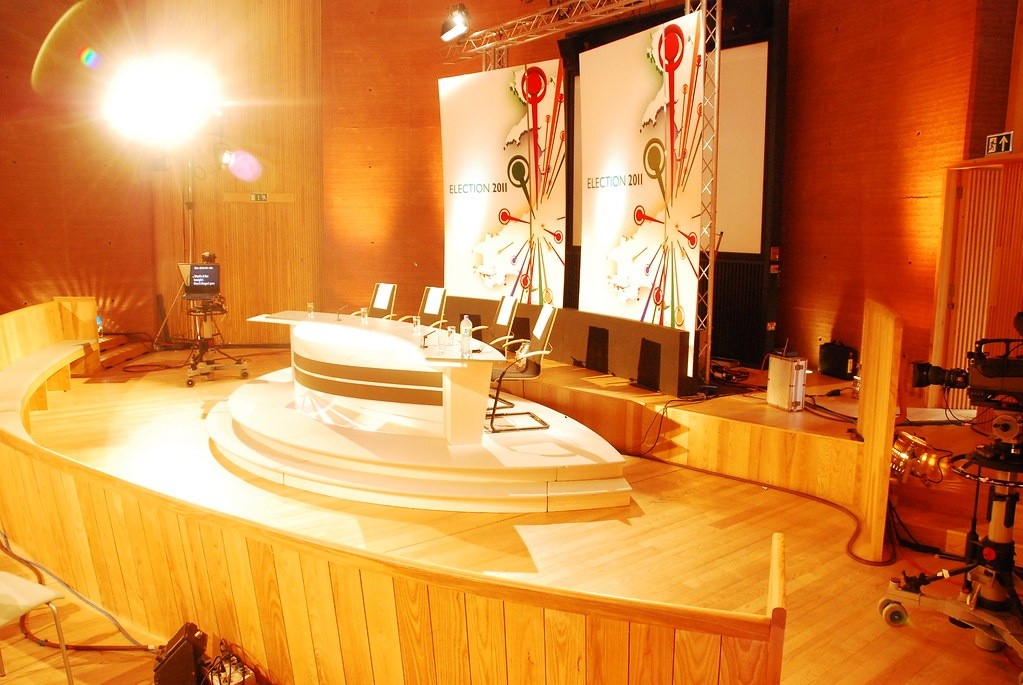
[459,315,473,357]
[97,315,103,340]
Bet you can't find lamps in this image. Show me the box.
[439,1,472,43]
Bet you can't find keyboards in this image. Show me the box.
[186,294,213,300]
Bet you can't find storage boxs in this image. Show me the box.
[766,351,808,412]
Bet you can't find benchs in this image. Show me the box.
[0,297,786,685]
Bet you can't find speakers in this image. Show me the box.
[819,343,858,381]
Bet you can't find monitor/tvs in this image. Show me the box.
[178,263,220,294]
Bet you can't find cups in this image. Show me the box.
[412,316,420,336]
[448,325,456,346]
[307,303,314,319]
[437,334,446,355]
[361,307,368,324]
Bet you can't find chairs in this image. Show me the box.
[0,569,78,685]
[346,281,398,321]
[485,301,558,434]
[394,285,451,333]
[463,295,522,410]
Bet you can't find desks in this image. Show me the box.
[244,313,506,446]
